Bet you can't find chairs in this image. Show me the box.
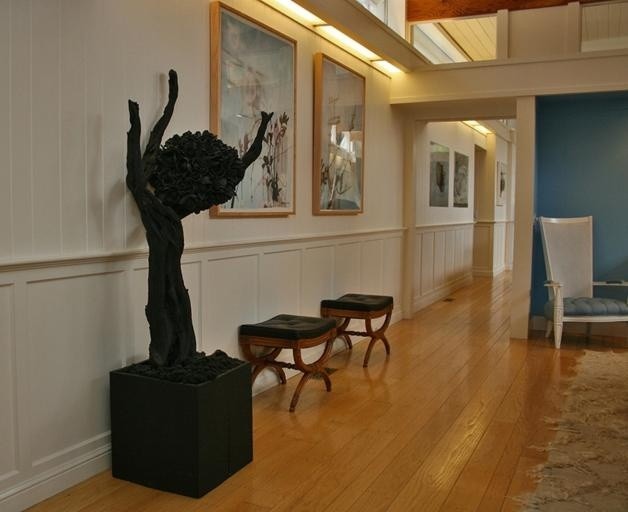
[537,215,628,349]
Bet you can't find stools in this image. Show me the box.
[240,314,337,412]
[321,294,393,367]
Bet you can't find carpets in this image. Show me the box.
[507,350,628,512]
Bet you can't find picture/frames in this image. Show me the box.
[209,0,299,218]
[313,51,368,216]
[454,151,469,207]
[496,161,508,206]
[429,141,451,208]
